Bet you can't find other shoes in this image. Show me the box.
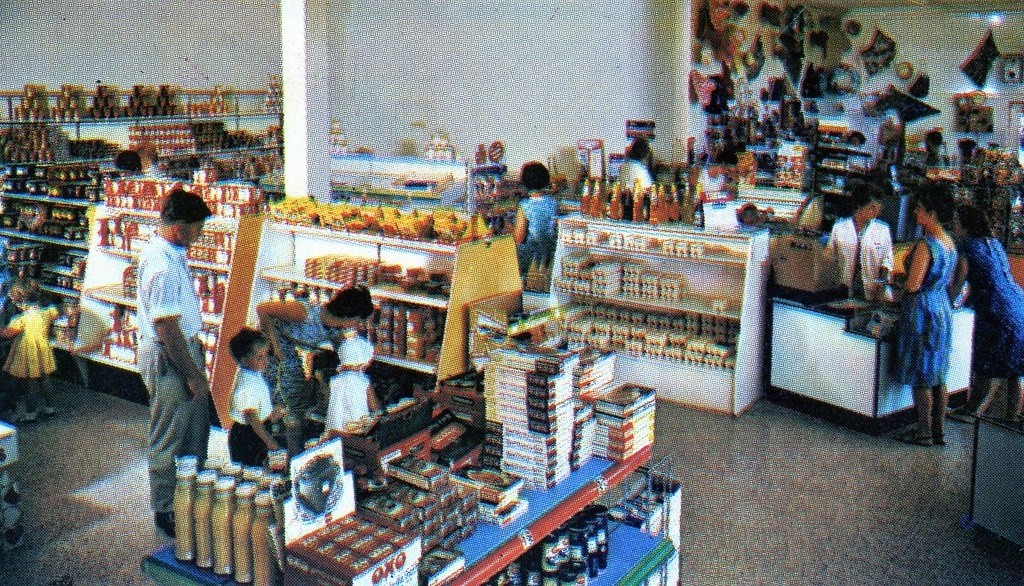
[948,404,978,423]
[1007,413,1023,427]
[37,409,57,421]
[16,416,40,426]
[154,511,177,538]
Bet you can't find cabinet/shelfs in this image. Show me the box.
[0,85,283,427]
[551,227,766,411]
[230,223,524,427]
[259,156,469,218]
[140,446,673,586]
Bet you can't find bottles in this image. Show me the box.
[233,467,285,586]
[174,455,196,559]
[583,179,695,225]
[194,456,241,574]
[193,152,282,183]
[489,505,608,586]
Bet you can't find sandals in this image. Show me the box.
[932,433,947,445]
[894,430,934,447]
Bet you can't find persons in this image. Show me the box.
[501,160,557,271]
[227,284,380,470]
[821,183,896,302]
[892,184,959,446]
[620,137,654,191]
[135,189,213,539]
[943,203,1024,426]
[0,279,58,424]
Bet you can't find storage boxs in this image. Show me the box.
[284,345,652,586]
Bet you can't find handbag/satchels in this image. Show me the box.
[953,280,971,308]
[866,310,902,340]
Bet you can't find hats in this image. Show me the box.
[334,330,373,376]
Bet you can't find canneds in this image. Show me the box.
[484,506,609,586]
[304,256,440,365]
[6,84,281,289]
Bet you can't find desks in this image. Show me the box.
[767,301,975,437]
